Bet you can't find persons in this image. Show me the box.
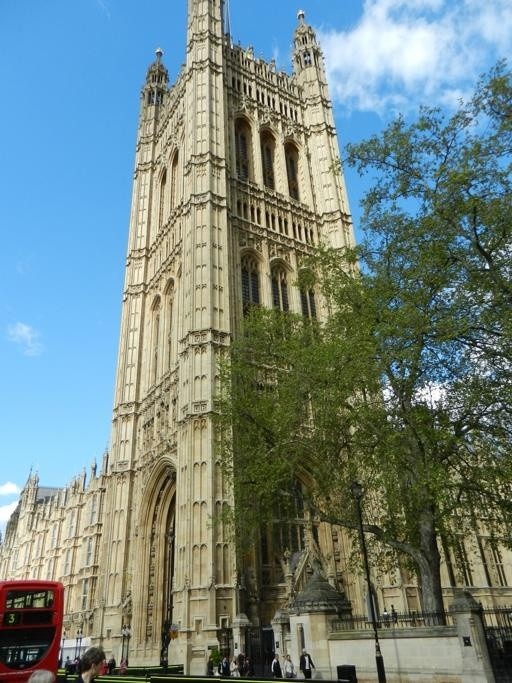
[26,669,56,683]
[390,604,399,627]
[217,652,256,678]
[63,643,128,683]
[383,607,389,628]
[271,653,282,678]
[283,655,297,678]
[299,647,315,679]
[207,656,215,675]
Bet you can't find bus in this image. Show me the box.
[0,580,65,682]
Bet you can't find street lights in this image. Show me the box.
[74,629,80,657]
[121,624,126,665]
[78,629,83,658]
[348,481,387,682]
[125,623,133,665]
[59,630,67,667]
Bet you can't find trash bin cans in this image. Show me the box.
[337,664,357,683]
